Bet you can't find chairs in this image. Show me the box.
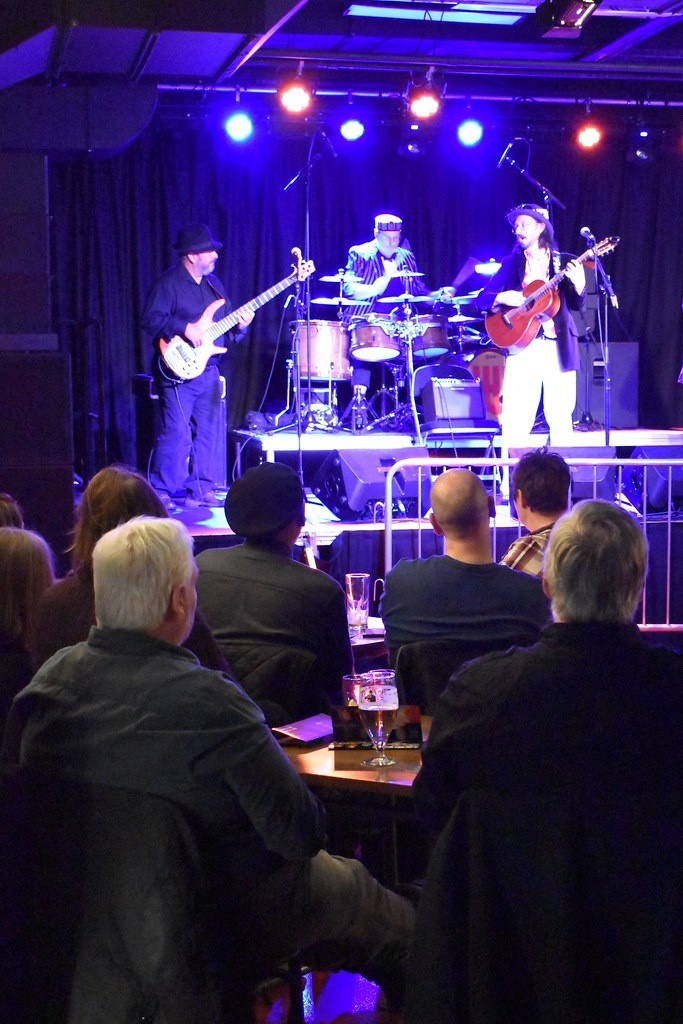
[214,643,324,729]
[408,361,504,511]
[392,636,538,743]
[1,783,317,1024]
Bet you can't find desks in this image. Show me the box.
[272,715,445,895]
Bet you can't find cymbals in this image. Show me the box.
[309,296,372,306]
[376,294,437,303]
[447,314,484,323]
[386,269,425,277]
[319,273,364,282]
[442,296,476,304]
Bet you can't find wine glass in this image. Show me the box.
[358,672,400,767]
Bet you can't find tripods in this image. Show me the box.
[573,324,618,430]
[267,145,422,436]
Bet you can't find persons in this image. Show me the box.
[476,203,587,446]
[500,448,575,580]
[0,517,413,1024]
[382,467,554,666]
[342,215,457,430]
[403,501,683,1024]
[0,491,54,696]
[29,465,233,682]
[140,221,255,507]
[194,460,354,728]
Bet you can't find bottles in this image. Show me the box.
[342,669,395,707]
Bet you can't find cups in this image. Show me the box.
[345,573,369,630]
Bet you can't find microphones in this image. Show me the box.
[431,291,444,313]
[291,247,302,260]
[496,138,516,169]
[292,296,308,313]
[318,129,337,158]
[580,227,596,240]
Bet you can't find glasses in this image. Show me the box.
[513,222,539,230]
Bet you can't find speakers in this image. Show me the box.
[571,341,639,430]
[422,377,486,422]
[622,445,683,515]
[508,446,617,519]
[310,446,433,521]
[0,351,74,582]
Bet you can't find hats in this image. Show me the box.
[375,213,402,231]
[224,463,304,538]
[507,204,554,244]
[170,221,223,255]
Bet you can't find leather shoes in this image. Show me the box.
[184,490,225,508]
[157,493,176,511]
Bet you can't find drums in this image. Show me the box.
[289,319,353,381]
[451,348,506,425]
[404,313,450,357]
[348,312,402,362]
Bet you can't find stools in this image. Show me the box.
[366,358,400,428]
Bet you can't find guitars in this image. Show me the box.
[484,235,621,352]
[156,246,316,381]
